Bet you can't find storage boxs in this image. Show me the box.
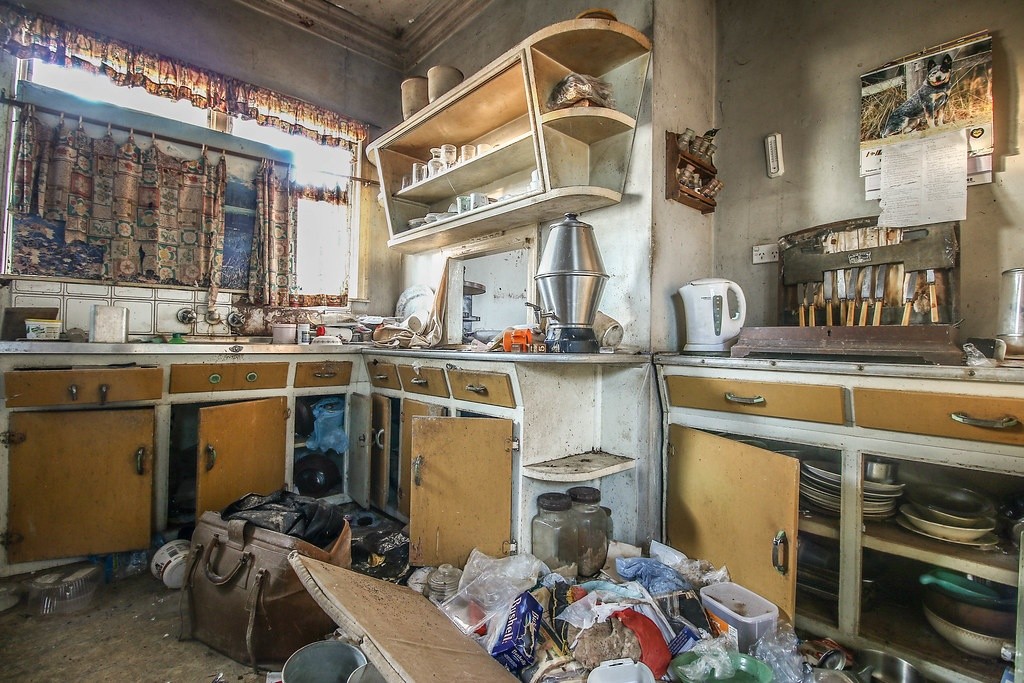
[491,591,544,670]
[700,582,778,654]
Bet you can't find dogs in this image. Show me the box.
[880,53,952,138]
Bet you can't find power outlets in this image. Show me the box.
[753,244,778,263]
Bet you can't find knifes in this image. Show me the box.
[901,272,918,326]
[797,284,805,326]
[846,268,860,326]
[837,269,846,326]
[926,269,939,322]
[859,266,873,326]
[808,283,815,327]
[872,265,886,326]
[824,271,833,326]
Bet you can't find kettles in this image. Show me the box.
[678,277,746,352]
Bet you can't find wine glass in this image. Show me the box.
[441,144,456,172]
[457,144,500,164]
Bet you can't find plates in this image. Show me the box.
[896,515,1001,545]
[799,460,906,521]
[408,202,458,228]
[326,327,352,343]
[395,285,434,323]
[672,650,773,682]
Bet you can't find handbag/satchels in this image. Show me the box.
[175,484,352,673]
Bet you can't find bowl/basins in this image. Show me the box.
[863,457,899,484]
[151,539,192,588]
[294,454,341,498]
[899,484,997,541]
[848,648,926,683]
[296,398,314,435]
[24,319,62,340]
[917,566,1018,662]
[312,335,343,345]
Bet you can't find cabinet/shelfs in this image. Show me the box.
[657,364,1024,683]
[666,129,717,214]
[366,18,652,255]
[0,353,650,577]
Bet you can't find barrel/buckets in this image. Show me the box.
[281,641,388,683]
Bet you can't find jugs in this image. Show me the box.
[801,648,874,683]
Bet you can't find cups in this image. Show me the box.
[456,192,488,214]
[402,162,428,190]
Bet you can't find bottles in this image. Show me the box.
[167,333,187,344]
[592,310,625,350]
[401,64,465,122]
[423,563,463,601]
[530,486,614,578]
[316,324,325,337]
[797,537,834,568]
[273,323,296,344]
[297,324,311,345]
[996,267,1024,354]
[678,128,717,163]
[427,147,444,179]
[342,288,349,307]
[677,164,724,200]
